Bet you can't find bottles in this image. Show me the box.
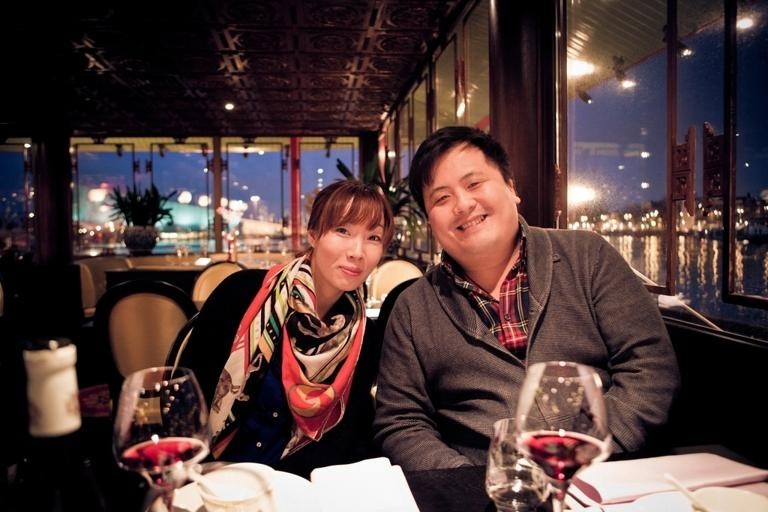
[13,335,119,512]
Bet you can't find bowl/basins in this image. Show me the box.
[690,487,767,512]
[196,462,277,512]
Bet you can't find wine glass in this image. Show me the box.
[514,362,614,512]
[112,365,209,512]
[170,224,286,266]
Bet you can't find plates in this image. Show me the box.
[633,492,693,512]
[168,471,312,512]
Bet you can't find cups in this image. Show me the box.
[485,420,548,512]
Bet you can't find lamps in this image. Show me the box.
[663,24,694,57]
[612,55,636,90]
[575,87,592,105]
[736,0,755,29]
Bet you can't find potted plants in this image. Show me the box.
[23,337,82,438]
[336,146,429,260]
[104,182,177,256]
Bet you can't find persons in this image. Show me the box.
[161,178,395,472]
[374,126,682,473]
